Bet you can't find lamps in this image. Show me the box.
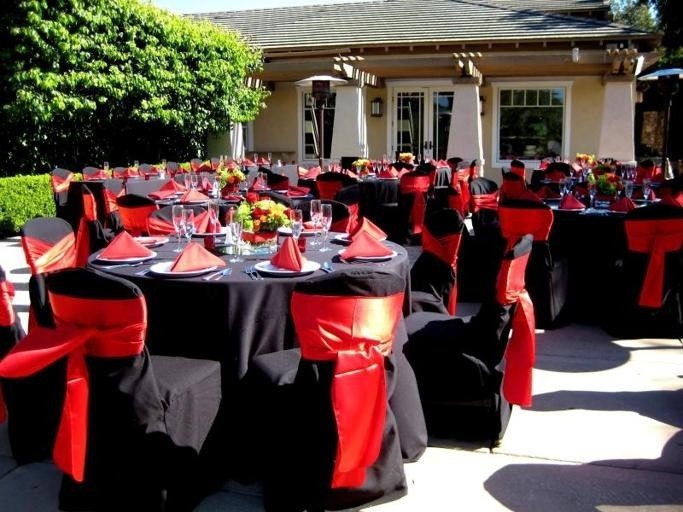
[367,96,385,120]
[478,94,487,117]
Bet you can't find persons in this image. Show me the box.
[501,144,519,159]
[537,134,561,156]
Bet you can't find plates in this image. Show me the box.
[338,247,396,261]
[335,233,387,244]
[254,260,320,275]
[149,262,215,277]
[130,236,169,247]
[286,195,307,198]
[96,250,158,261]
[274,190,288,194]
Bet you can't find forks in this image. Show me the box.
[243,263,264,281]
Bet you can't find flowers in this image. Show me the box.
[397,152,417,165]
[215,164,247,186]
[574,155,624,193]
[235,198,292,232]
[352,158,370,170]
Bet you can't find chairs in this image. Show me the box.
[1,153,682,507]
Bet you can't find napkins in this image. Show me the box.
[253,179,268,190]
[532,181,681,213]
[147,190,176,200]
[284,185,310,197]
[181,188,210,203]
[95,225,159,259]
[159,177,181,192]
[191,209,224,233]
[347,217,389,242]
[169,241,228,272]
[340,231,393,257]
[272,235,304,273]
[302,158,450,179]
[88,153,274,180]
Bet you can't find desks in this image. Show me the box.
[85,229,412,472]
[505,193,683,320]
[316,166,453,221]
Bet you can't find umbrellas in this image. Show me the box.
[636,67,682,161]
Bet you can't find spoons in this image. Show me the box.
[102,262,143,270]
[323,260,335,272]
[214,267,232,281]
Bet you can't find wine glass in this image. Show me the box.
[103,151,282,200]
[171,199,334,262]
[327,154,429,182]
[556,157,652,214]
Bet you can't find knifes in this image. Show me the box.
[202,267,229,281]
